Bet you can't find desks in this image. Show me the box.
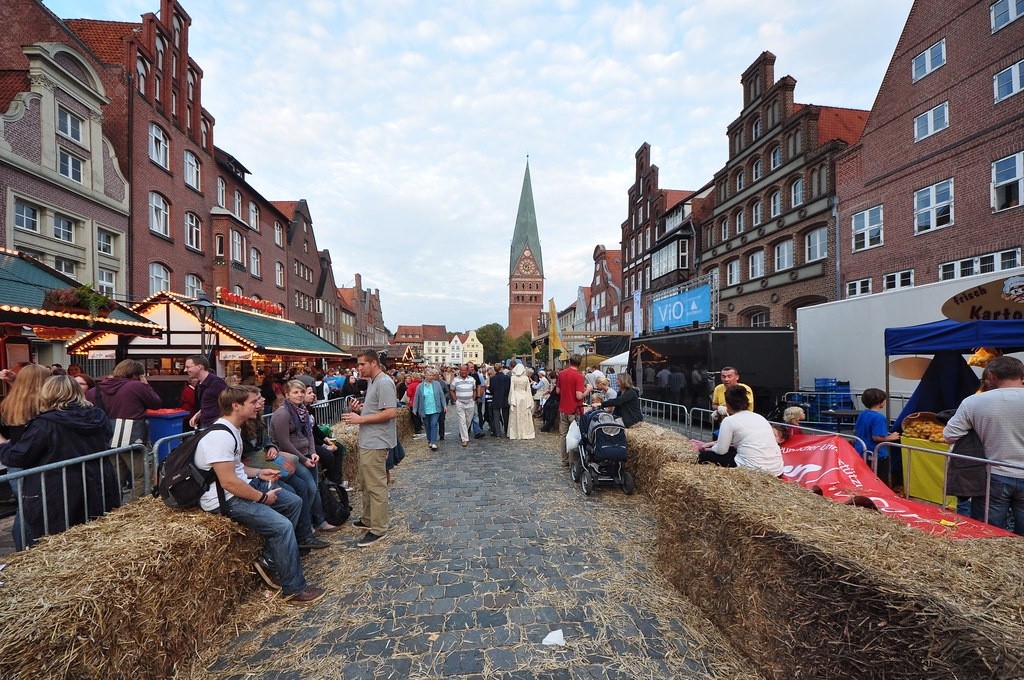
[900,435,958,511]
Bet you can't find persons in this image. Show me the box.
[942,356,1024,535]
[0,358,164,553]
[175,355,228,441]
[240,361,615,451]
[556,354,643,467]
[644,363,712,415]
[696,366,806,478]
[193,353,398,604]
[854,388,900,483]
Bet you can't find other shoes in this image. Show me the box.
[297,537,330,548]
[413,434,421,439]
[440,436,444,441]
[429,443,432,447]
[431,444,437,451]
[319,526,340,533]
[475,432,484,439]
[462,441,468,446]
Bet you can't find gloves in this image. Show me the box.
[711,411,717,420]
[717,406,728,416]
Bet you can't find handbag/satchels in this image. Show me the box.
[105,419,147,450]
[240,447,298,481]
[565,420,582,453]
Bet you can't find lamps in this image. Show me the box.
[642,330,647,336]
[665,326,669,332]
[544,337,549,345]
[530,341,537,349]
[693,321,699,328]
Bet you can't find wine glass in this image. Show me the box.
[328,433,337,451]
[342,407,353,429]
[264,478,276,507]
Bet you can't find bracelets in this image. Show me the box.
[258,493,268,504]
[255,469,259,478]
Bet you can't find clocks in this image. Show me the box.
[518,258,537,276]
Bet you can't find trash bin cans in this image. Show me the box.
[146,408,190,464]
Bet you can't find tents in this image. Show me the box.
[885,315,1024,488]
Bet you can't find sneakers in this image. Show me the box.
[352,519,370,530]
[287,586,327,604]
[358,532,386,546]
[254,559,281,589]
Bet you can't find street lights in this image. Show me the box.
[188,289,216,365]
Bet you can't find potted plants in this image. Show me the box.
[41,282,120,329]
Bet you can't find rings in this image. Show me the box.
[273,454,277,459]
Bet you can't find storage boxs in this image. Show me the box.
[791,377,855,431]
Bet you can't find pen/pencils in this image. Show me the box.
[267,480,272,489]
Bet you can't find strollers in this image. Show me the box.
[567,406,635,496]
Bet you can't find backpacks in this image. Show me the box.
[318,481,349,525]
[156,423,237,510]
